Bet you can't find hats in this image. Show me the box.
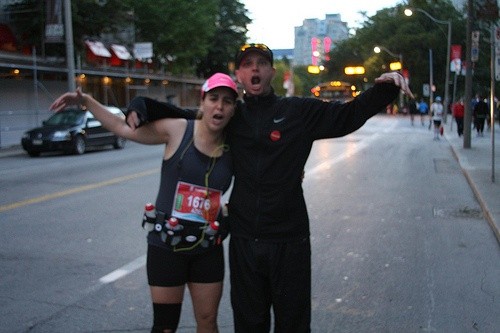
[235,43,273,66]
[201,73,240,101]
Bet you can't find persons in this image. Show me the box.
[50,72,238,333]
[418,98,428,126]
[408,93,418,125]
[429,96,444,139]
[125,42,416,333]
[469,94,498,135]
[453,98,466,136]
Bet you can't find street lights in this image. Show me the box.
[374,46,403,74]
[404,8,451,124]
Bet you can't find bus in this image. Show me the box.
[320,80,352,104]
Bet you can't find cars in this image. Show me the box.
[21,107,126,157]
